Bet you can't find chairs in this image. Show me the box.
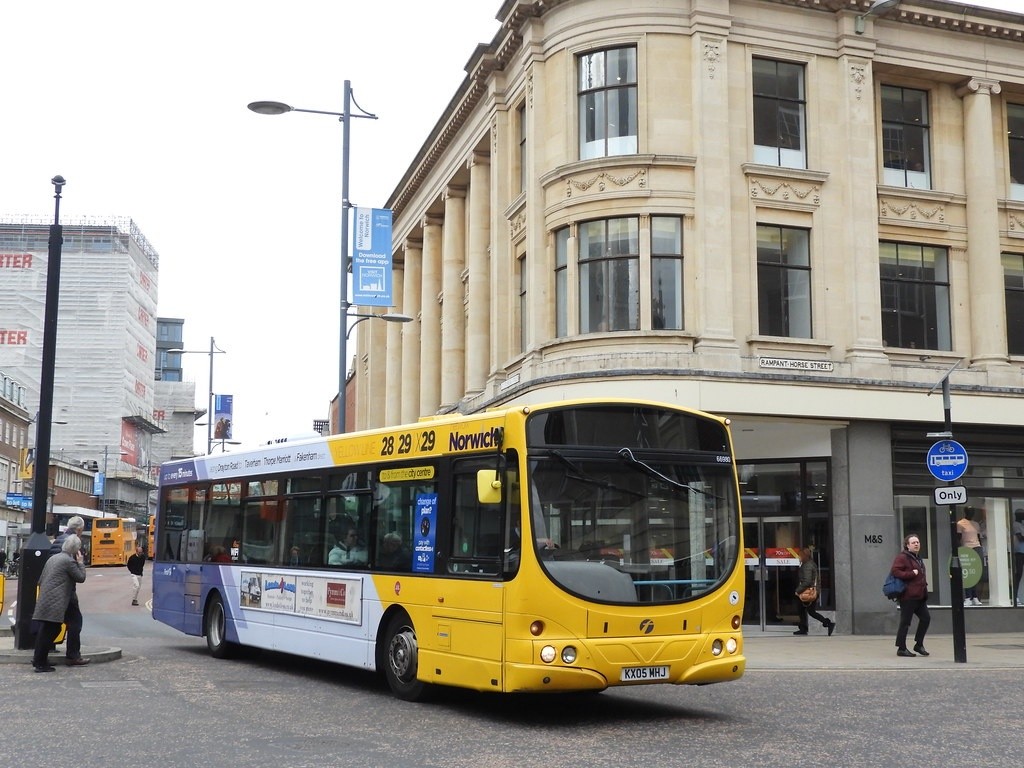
[242,541,502,581]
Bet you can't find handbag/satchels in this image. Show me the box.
[883,567,906,602]
[799,586,817,601]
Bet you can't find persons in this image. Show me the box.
[127,546,146,606]
[891,533,931,656]
[31,515,91,673]
[0,547,19,572]
[793,547,836,636]
[212,536,239,563]
[383,533,411,572]
[328,519,373,570]
[956,505,985,606]
[499,512,554,575]
[1011,508,1024,606]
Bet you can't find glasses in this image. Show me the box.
[350,534,359,537]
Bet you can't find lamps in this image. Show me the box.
[855,0,900,35]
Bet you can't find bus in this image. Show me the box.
[152,397,747,703]
[148,516,156,558]
[91,518,138,566]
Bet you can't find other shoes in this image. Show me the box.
[828,623,836,636]
[34,666,56,672]
[67,657,91,666]
[132,600,139,605]
[973,597,982,605]
[964,598,971,606]
[793,630,808,636]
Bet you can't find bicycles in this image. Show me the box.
[3,561,20,578]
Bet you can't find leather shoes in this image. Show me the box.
[897,648,916,657]
[914,644,929,655]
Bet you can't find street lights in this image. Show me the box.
[926,359,967,663]
[166,337,241,455]
[248,80,414,434]
[89,445,129,518]
[195,423,224,453]
[14,175,66,650]
